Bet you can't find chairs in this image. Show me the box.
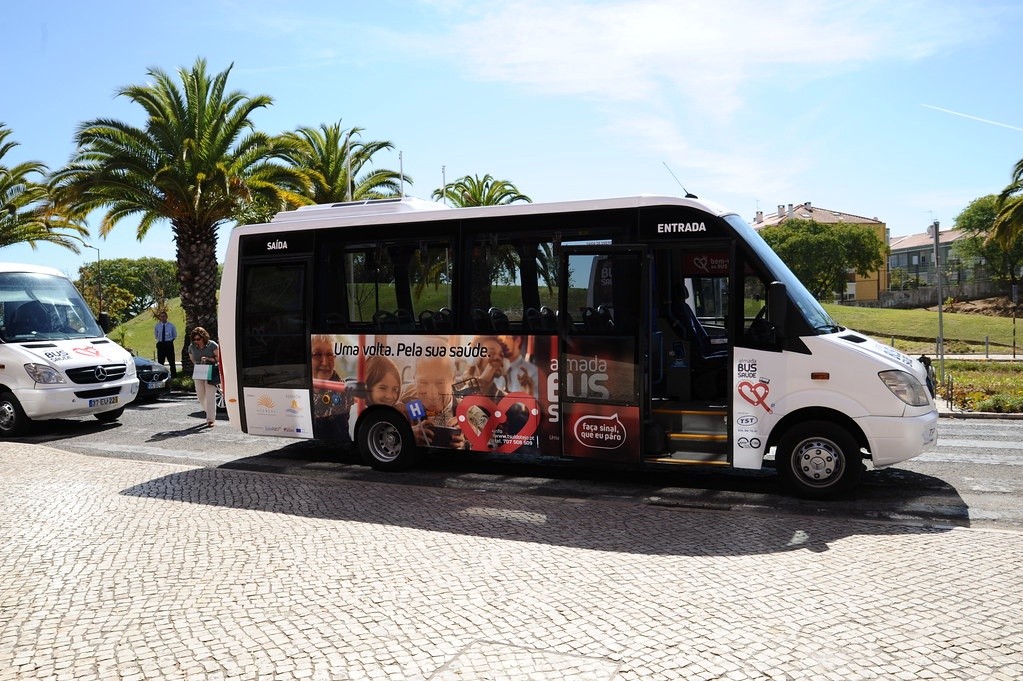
[487,306,512,330]
[597,305,615,329]
[581,307,608,334]
[540,306,558,331]
[472,309,497,331]
[524,308,547,332]
[665,280,729,378]
[322,308,454,331]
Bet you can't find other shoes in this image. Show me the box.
[208,422,214,427]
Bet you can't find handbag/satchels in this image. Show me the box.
[207,362,220,385]
[192,361,212,381]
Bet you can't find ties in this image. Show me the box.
[162,325,165,343]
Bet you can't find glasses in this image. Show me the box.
[192,337,202,341]
[312,352,336,361]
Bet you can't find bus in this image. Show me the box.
[217,193,939,503]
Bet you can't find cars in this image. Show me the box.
[132,355,170,403]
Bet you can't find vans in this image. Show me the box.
[0,260,139,437]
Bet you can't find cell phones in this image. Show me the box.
[425,425,462,447]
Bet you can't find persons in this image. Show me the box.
[188,327,220,427]
[311,334,555,449]
[155,311,177,379]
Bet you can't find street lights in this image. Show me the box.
[84,243,102,314]
